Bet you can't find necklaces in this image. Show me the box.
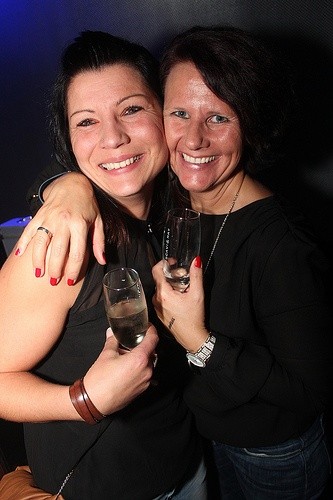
[180,173,244,292]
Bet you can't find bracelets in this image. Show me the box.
[68,379,106,425]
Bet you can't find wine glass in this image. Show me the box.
[102,267,157,371]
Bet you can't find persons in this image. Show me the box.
[13,28,333,500]
[0,30,207,500]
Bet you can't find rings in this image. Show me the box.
[151,354,159,368]
[38,226,51,238]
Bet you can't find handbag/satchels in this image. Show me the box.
[0,464,67,500]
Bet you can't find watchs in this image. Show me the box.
[187,334,217,367]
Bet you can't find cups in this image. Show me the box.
[161,207,200,295]
